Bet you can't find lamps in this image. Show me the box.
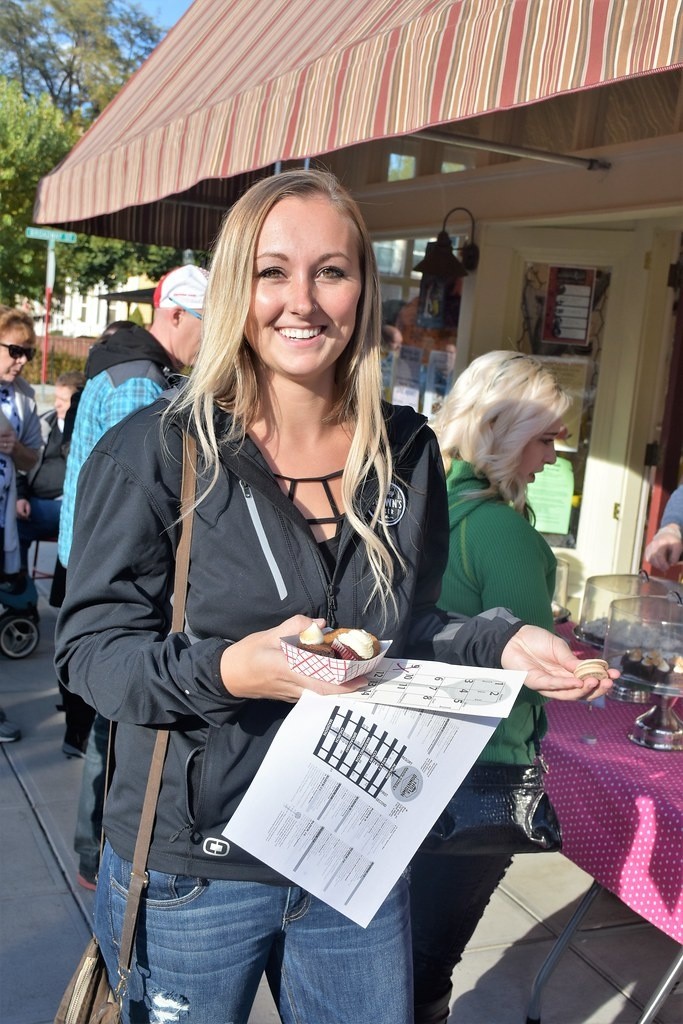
[411,206,480,278]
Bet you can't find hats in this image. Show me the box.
[153,264,210,310]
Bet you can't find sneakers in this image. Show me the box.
[0,710,21,742]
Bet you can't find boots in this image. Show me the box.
[414,981,453,1024]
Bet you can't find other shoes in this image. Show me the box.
[63,728,90,759]
[78,868,99,891]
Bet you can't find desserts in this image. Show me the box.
[621,650,683,686]
[297,622,380,662]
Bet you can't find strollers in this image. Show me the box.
[0,568,41,661]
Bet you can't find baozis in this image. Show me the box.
[584,617,680,648]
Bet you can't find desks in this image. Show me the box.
[525,622,683,1024]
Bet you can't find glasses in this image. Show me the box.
[0,343,37,362]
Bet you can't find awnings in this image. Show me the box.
[33,0,683,252]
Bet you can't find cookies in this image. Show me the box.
[573,660,609,678]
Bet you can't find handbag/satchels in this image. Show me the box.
[53,938,124,1024]
[415,765,562,859]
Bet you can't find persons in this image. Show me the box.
[53,163,620,1024]
[0,266,683,1024]
